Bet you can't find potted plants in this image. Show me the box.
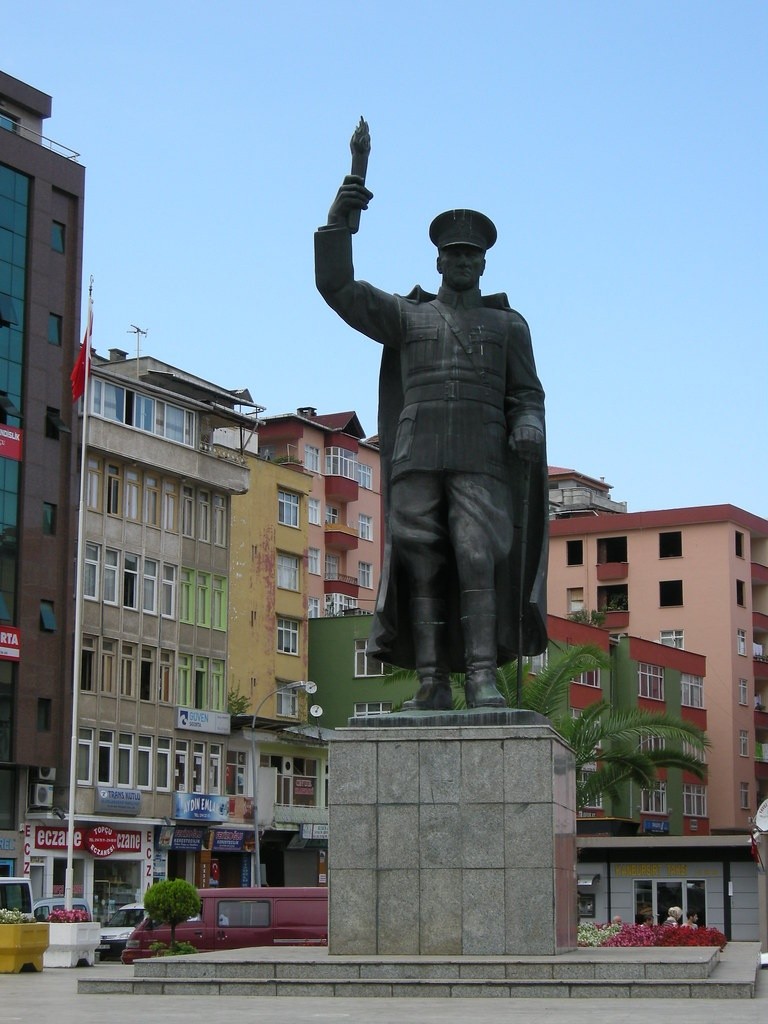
[762,655,766,661]
[272,455,302,471]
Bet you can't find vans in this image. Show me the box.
[34,898,93,922]
[121,887,327,965]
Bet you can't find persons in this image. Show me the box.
[615,906,699,930]
[312,176,550,711]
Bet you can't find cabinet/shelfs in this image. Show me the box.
[98,882,136,924]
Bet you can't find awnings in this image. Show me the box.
[148,369,266,411]
[578,873,600,887]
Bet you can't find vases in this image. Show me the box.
[0,924,50,974]
[37,922,101,967]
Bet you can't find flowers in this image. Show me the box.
[47,908,91,923]
[0,907,37,924]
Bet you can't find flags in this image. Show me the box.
[750,835,760,864]
[69,310,96,404]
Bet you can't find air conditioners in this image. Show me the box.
[32,765,56,780]
[29,783,53,807]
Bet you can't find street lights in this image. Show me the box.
[250,681,307,883]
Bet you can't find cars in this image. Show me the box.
[96,901,229,956]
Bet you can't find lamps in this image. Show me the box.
[52,807,66,820]
